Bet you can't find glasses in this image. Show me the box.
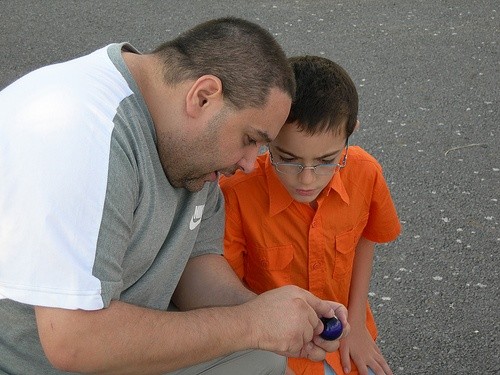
[267,137,349,174]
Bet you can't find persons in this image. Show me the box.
[1,16,352,375]
[218,55,401,375]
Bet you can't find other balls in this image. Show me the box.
[318,314,343,341]
